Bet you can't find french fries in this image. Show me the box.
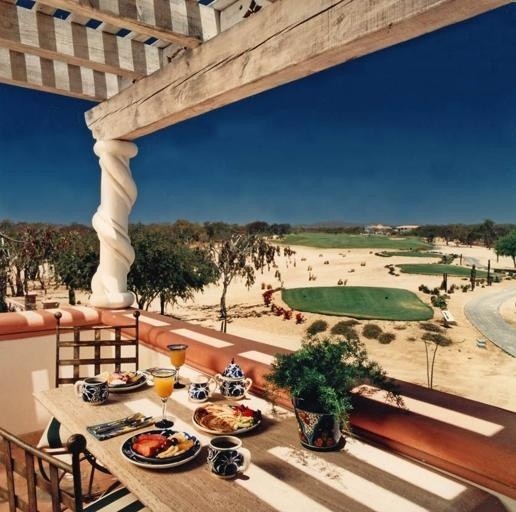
[205,404,253,430]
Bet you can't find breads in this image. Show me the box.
[193,408,235,433]
[130,433,166,457]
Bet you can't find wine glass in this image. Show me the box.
[151,368,177,428]
[166,343,188,389]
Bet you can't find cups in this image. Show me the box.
[74,377,109,406]
[187,376,218,403]
[207,435,251,480]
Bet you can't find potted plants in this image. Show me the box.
[263,332,406,452]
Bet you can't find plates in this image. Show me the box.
[98,372,149,393]
[192,409,262,436]
[120,430,203,469]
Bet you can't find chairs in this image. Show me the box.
[0,311,145,511]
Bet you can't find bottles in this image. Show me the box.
[214,364,253,401]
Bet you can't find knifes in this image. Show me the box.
[93,415,153,435]
